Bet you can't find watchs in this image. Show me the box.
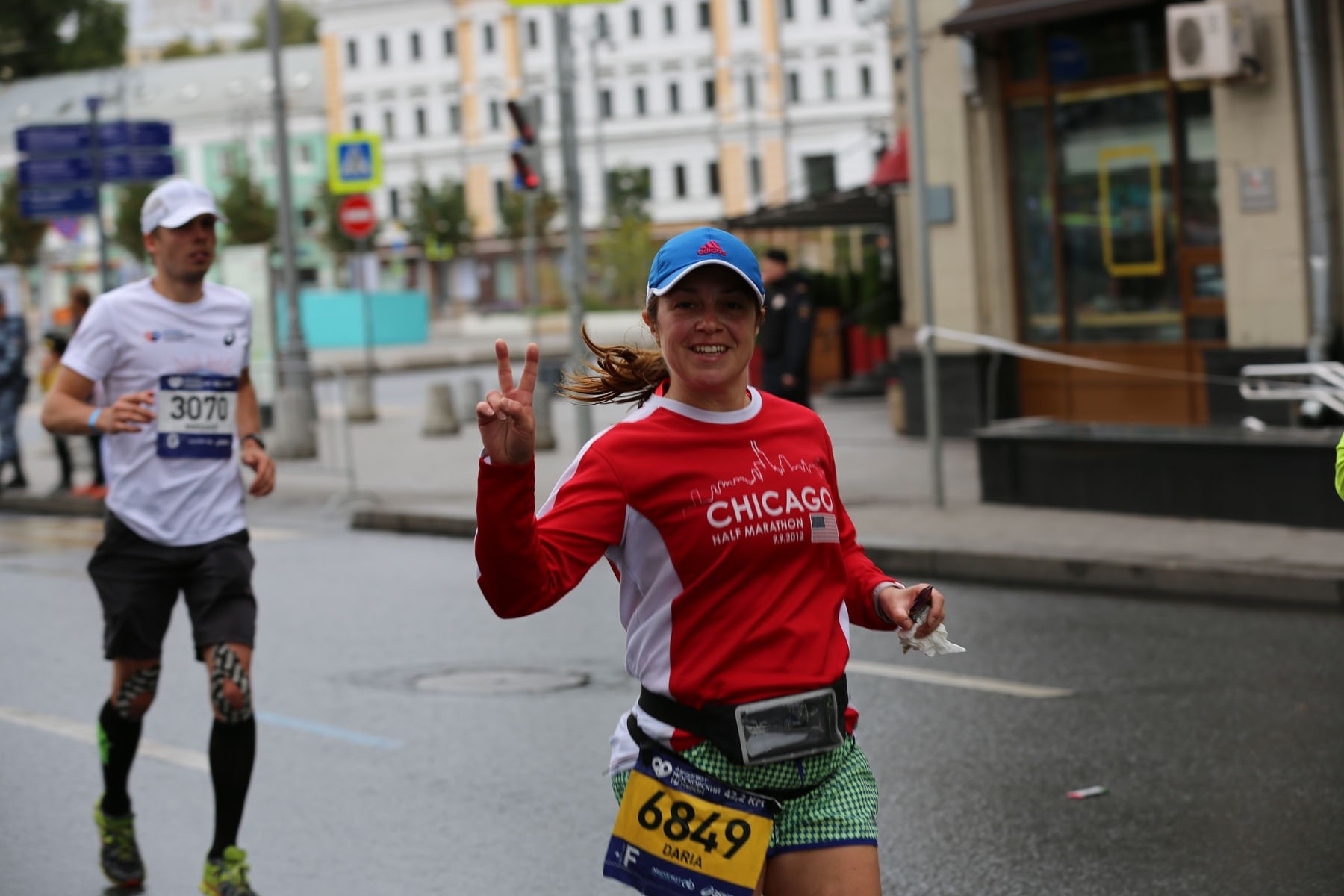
[240,432,265,450]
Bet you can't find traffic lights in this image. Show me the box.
[506,96,543,192]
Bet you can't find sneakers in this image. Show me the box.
[94,790,147,887]
[199,846,257,896]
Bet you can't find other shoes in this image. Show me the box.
[7,474,27,487]
[73,482,106,498]
[56,479,73,490]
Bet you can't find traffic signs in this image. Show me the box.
[11,115,178,215]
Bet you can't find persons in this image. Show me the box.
[0,290,30,492]
[39,179,274,895]
[45,289,106,497]
[474,227,946,895]
[751,244,811,411]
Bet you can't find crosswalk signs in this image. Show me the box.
[324,134,383,195]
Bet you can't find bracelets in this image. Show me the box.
[87,405,102,434]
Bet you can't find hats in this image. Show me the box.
[142,180,230,235]
[647,227,765,306]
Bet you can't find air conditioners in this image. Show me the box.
[1165,1,1255,80]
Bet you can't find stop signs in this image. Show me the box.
[336,193,378,242]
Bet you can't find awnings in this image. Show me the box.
[943,0,1150,51]
